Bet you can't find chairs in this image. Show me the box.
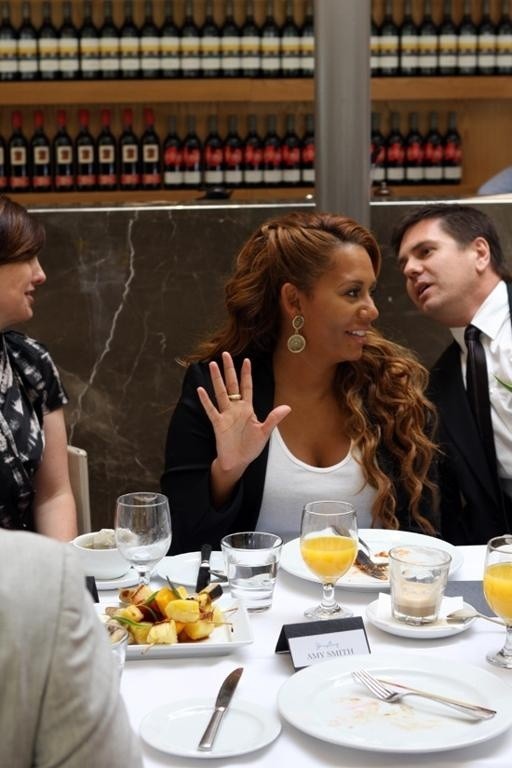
[62,443,90,541]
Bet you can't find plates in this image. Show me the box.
[139,700,281,759]
[280,528,463,589]
[159,549,230,586]
[95,599,253,657]
[90,567,139,591]
[276,653,512,753]
[366,593,479,640]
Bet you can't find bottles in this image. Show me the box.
[370,0,512,78]
[370,109,464,188]
[1,105,314,188]
[0,1,315,81]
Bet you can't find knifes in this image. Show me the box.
[195,543,213,594]
[198,667,245,751]
[331,524,387,580]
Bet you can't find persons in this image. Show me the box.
[160,210,442,556]
[389,202,512,547]
[1,192,79,545]
[0,529,142,768]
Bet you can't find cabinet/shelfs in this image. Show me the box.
[0,75,315,214]
[369,73,512,207]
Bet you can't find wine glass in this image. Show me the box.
[115,492,172,582]
[482,534,512,668]
[300,501,358,621]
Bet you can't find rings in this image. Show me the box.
[228,394,241,401]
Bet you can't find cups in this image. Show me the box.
[388,543,453,625]
[74,531,130,578]
[221,531,282,612]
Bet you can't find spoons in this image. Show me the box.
[448,606,508,628]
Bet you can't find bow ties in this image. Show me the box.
[464,325,497,478]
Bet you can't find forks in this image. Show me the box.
[354,669,494,720]
